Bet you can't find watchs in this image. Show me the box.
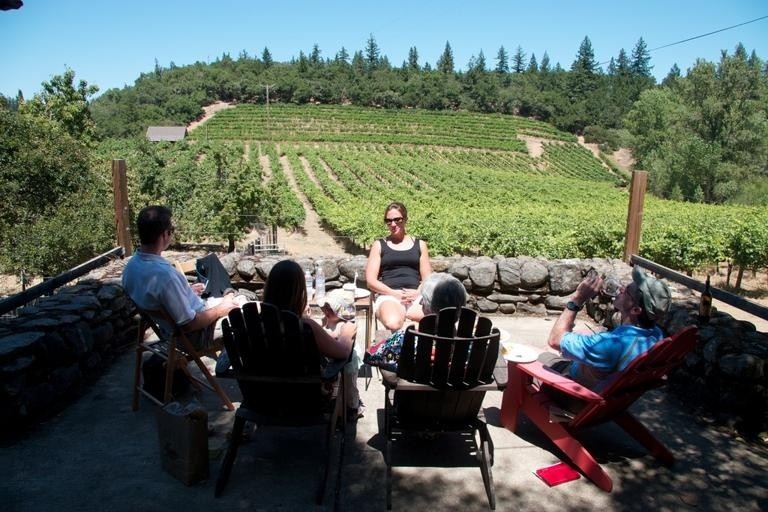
[565,300,584,312]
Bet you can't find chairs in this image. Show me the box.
[377,305,501,512]
[174,253,232,298]
[214,304,355,512]
[132,300,235,411]
[501,325,701,491]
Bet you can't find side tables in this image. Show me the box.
[305,288,373,355]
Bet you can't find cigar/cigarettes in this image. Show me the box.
[203,280,210,291]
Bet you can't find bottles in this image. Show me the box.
[304,267,313,301]
[314,267,326,298]
[340,282,356,319]
[698,273,713,323]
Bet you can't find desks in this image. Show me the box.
[223,252,292,289]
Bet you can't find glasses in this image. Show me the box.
[384,218,405,225]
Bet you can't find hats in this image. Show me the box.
[632,264,670,319]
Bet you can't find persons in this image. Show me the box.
[216,261,364,424]
[537,262,669,396]
[364,272,502,372]
[365,202,433,330]
[122,205,253,354]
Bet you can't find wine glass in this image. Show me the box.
[585,270,624,298]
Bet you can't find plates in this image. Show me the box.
[329,287,371,300]
[502,351,537,364]
